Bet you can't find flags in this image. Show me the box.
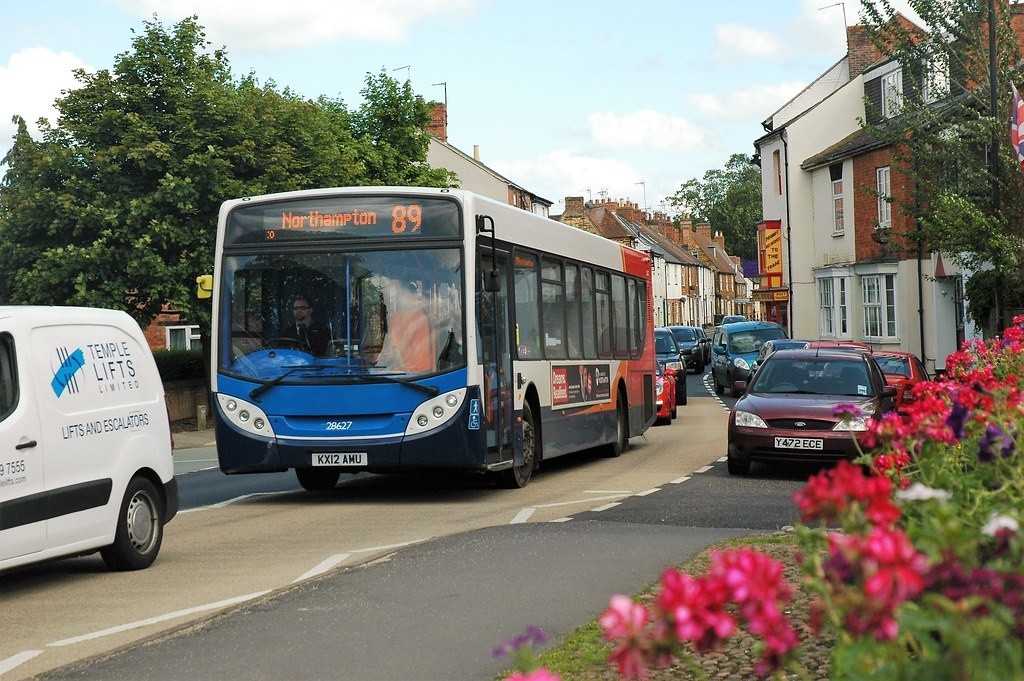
[1011,84,1024,169]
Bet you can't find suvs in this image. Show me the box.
[654,327,692,405]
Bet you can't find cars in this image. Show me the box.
[664,326,707,374]
[745,339,809,388]
[727,349,898,476]
[695,327,712,366]
[600,348,677,426]
[802,341,873,352]
[721,315,748,325]
[856,350,931,415]
[711,321,813,397]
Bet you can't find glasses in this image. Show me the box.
[295,306,311,311]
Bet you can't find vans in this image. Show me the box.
[0,304,180,573]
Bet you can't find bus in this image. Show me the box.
[195,186,658,493]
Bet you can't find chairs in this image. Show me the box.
[481,320,636,363]
[841,367,866,390]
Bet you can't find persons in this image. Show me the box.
[390,285,437,373]
[279,297,331,357]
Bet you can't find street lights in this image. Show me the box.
[393,64,411,81]
[431,81,448,127]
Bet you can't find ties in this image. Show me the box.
[298,323,307,349]
